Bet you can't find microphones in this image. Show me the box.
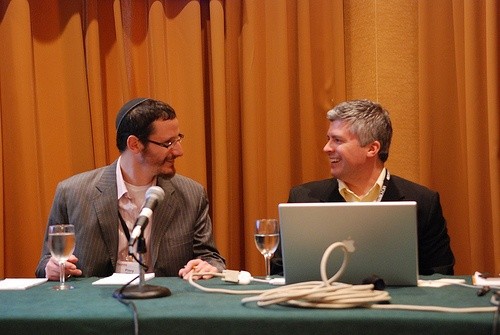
[128,185,165,254]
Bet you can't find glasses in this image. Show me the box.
[141,134,184,149]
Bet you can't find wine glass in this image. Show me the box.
[47,224,75,292]
[254,218,280,282]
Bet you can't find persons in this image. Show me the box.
[270,99,456,276]
[35,97,227,280]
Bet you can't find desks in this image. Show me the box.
[0,273,500,335]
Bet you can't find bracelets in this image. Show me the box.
[67,274,71,278]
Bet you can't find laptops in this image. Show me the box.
[278,202,420,287]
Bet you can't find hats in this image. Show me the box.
[116,98,148,134]
